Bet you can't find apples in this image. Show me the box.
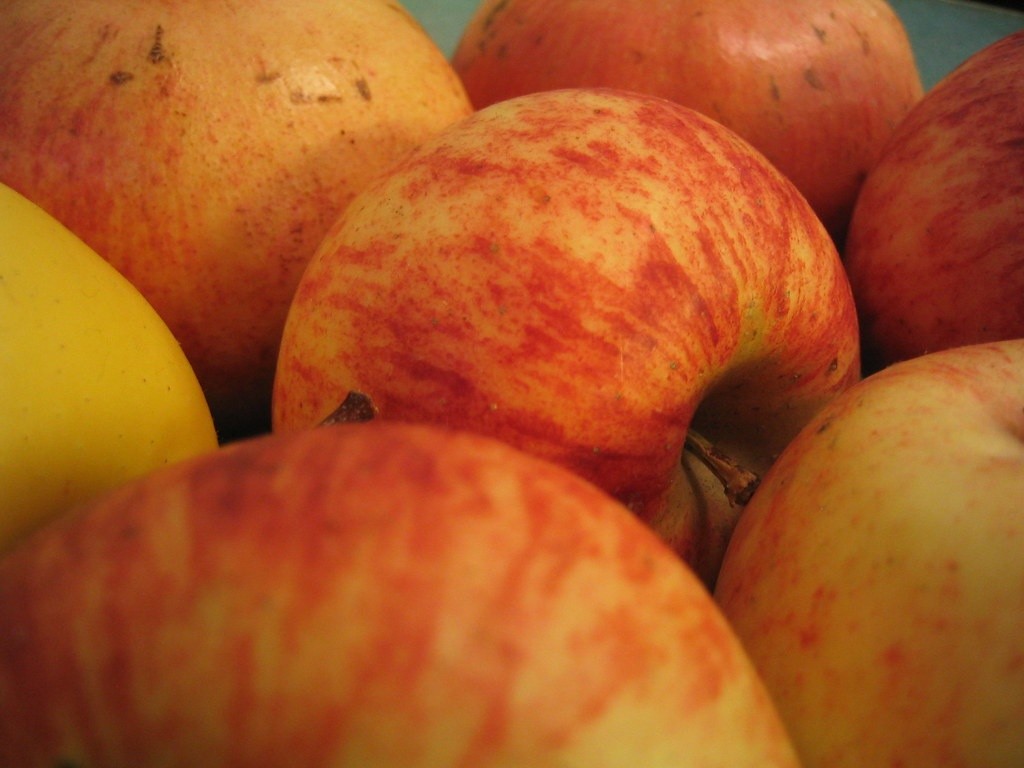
[449,1,927,258]
[837,31,1023,376]
[274,89,862,596]
[0,421,811,768]
[0,0,476,440]
[709,334,1024,768]
